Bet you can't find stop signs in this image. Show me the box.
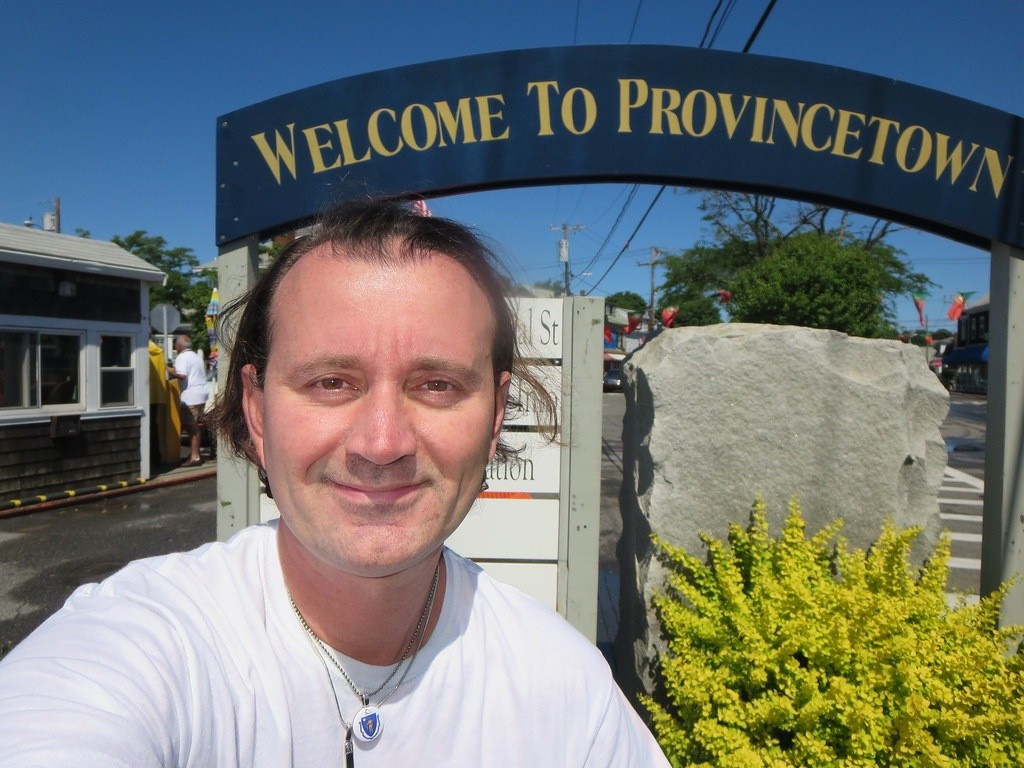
[929,360,942,368]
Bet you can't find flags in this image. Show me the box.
[946,289,978,321]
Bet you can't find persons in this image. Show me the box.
[148,324,169,476]
[165,333,211,467]
[0,187,675,767]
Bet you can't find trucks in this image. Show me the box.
[153,333,205,381]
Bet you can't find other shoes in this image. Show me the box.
[181,458,202,467]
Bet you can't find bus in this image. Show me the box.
[943,297,991,395]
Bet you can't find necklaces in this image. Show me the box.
[276,555,439,768]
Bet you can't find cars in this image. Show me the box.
[603,369,623,392]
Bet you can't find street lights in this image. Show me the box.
[564,273,594,300]
